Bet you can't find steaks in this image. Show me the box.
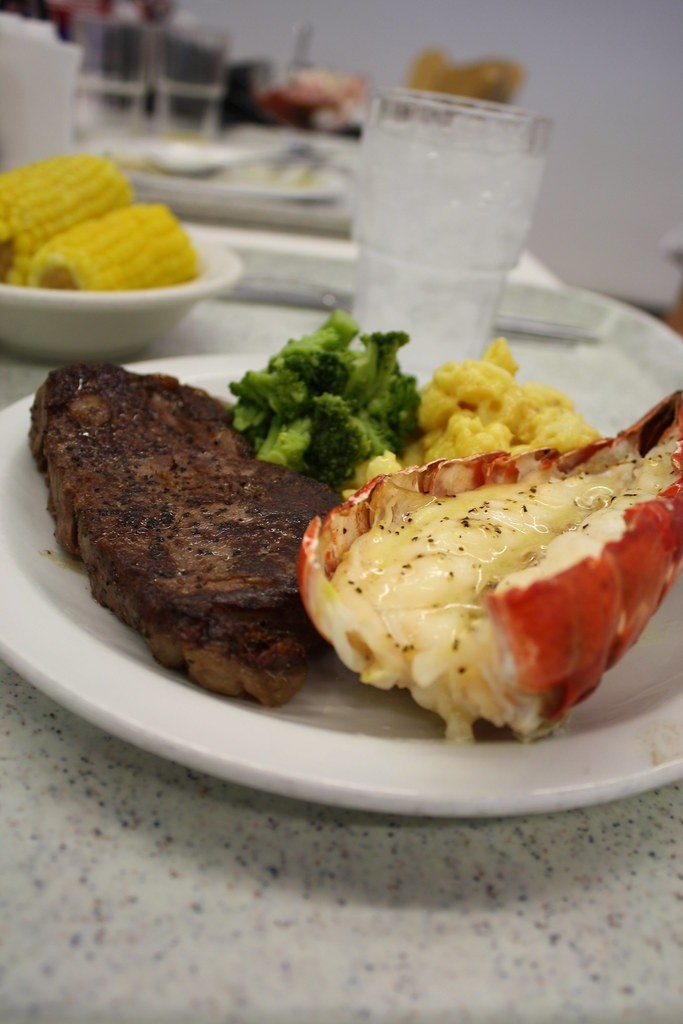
[29,362,353,704]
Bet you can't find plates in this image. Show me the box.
[86,127,371,228]
[1,353,683,817]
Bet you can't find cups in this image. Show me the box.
[74,11,151,148]
[156,12,232,149]
[355,88,557,387]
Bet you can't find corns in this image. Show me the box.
[0,153,199,292]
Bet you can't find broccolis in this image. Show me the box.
[232,309,420,488]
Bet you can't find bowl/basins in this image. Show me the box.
[0,233,243,362]
